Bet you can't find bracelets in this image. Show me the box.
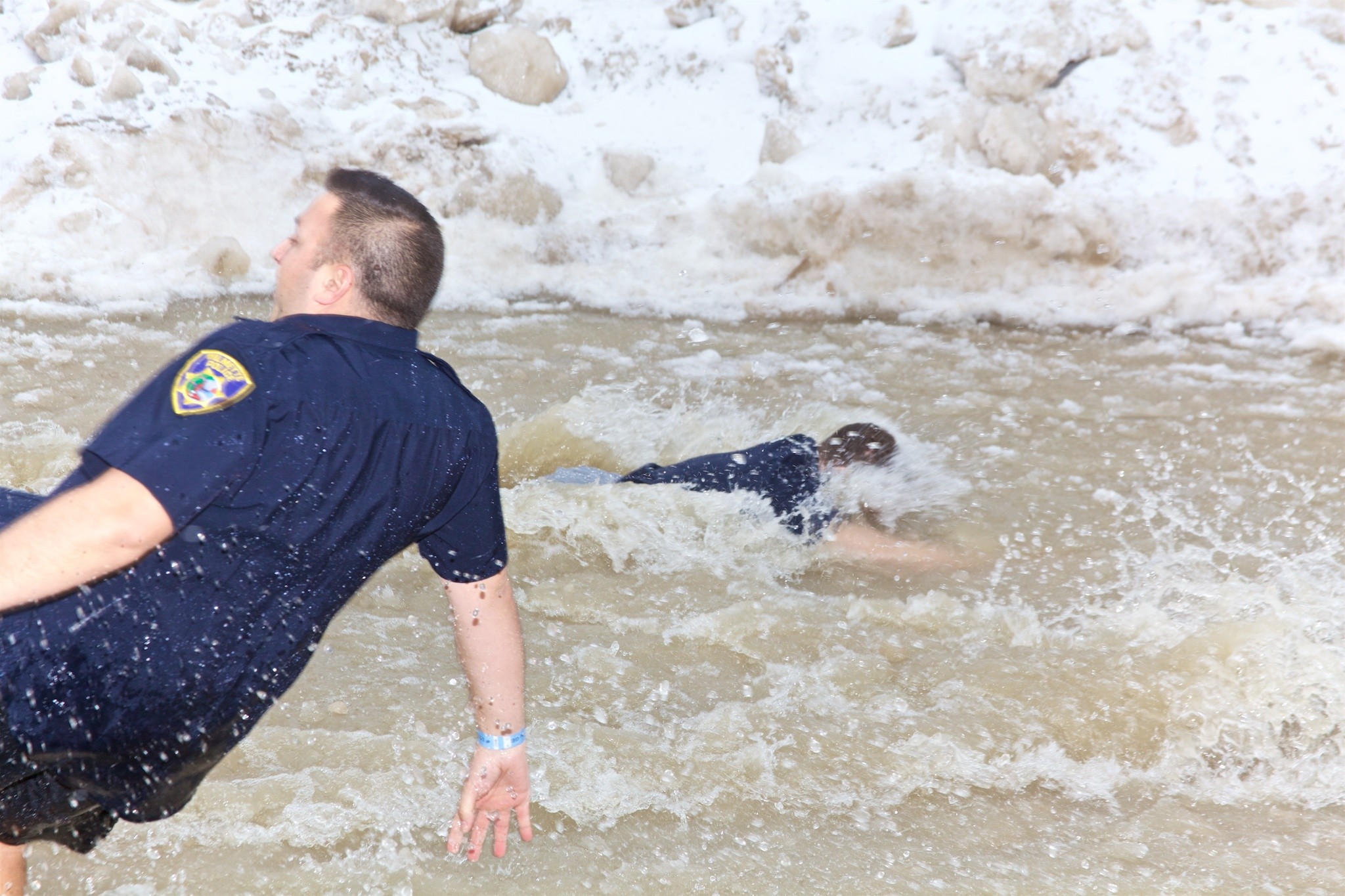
[477,727,527,750]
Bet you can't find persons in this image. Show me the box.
[552,423,938,560]
[0,166,534,896]
